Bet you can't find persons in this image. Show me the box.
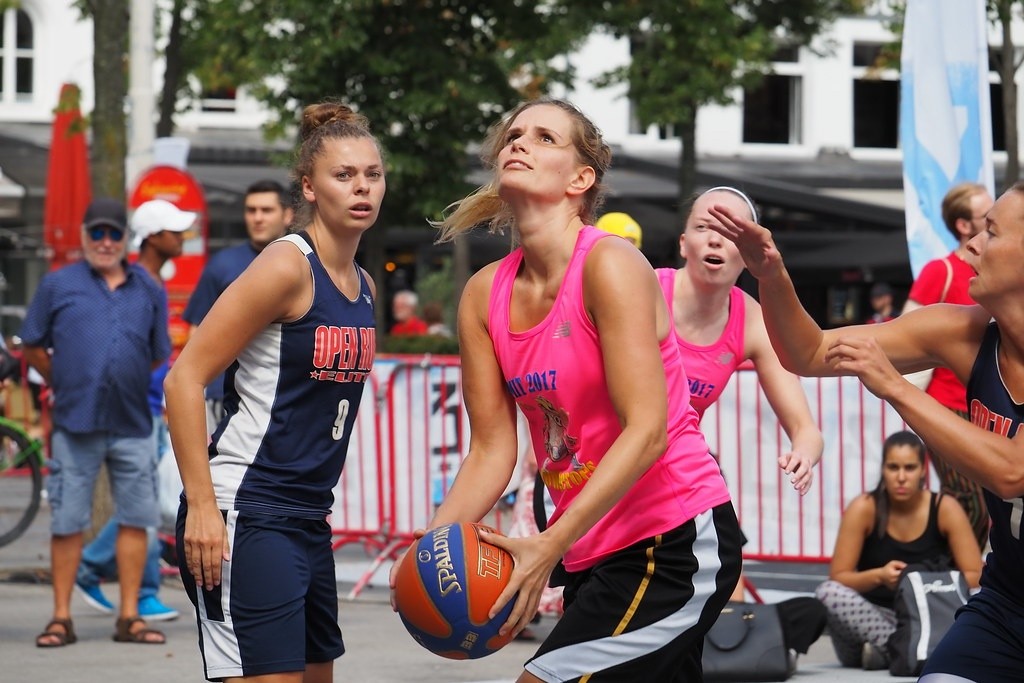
[17,197,195,649]
[390,98,1024,683]
[162,103,386,683]
[182,181,294,432]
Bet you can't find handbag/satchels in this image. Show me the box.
[700,596,789,683]
[888,565,972,676]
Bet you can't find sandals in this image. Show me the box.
[37,617,78,646]
[113,614,165,644]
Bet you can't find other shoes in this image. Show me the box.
[514,626,537,642]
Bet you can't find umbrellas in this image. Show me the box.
[45,84,90,271]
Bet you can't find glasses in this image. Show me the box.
[85,227,123,243]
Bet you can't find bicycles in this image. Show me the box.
[0,414,46,550]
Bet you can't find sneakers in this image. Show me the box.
[72,570,116,615]
[137,594,177,621]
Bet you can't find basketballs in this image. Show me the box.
[395,521,523,661]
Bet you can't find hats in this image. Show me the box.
[80,198,127,232]
[130,200,196,246]
[595,212,643,249]
